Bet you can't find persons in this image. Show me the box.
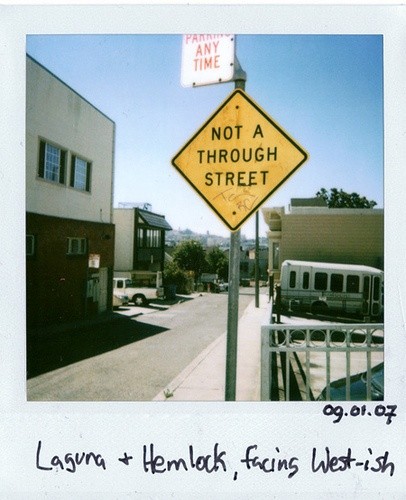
[266,269,275,304]
[48,272,74,326]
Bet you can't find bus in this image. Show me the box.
[280,260,383,317]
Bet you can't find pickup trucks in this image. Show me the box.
[113,278,166,306]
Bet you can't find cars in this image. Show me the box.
[316,362,383,400]
